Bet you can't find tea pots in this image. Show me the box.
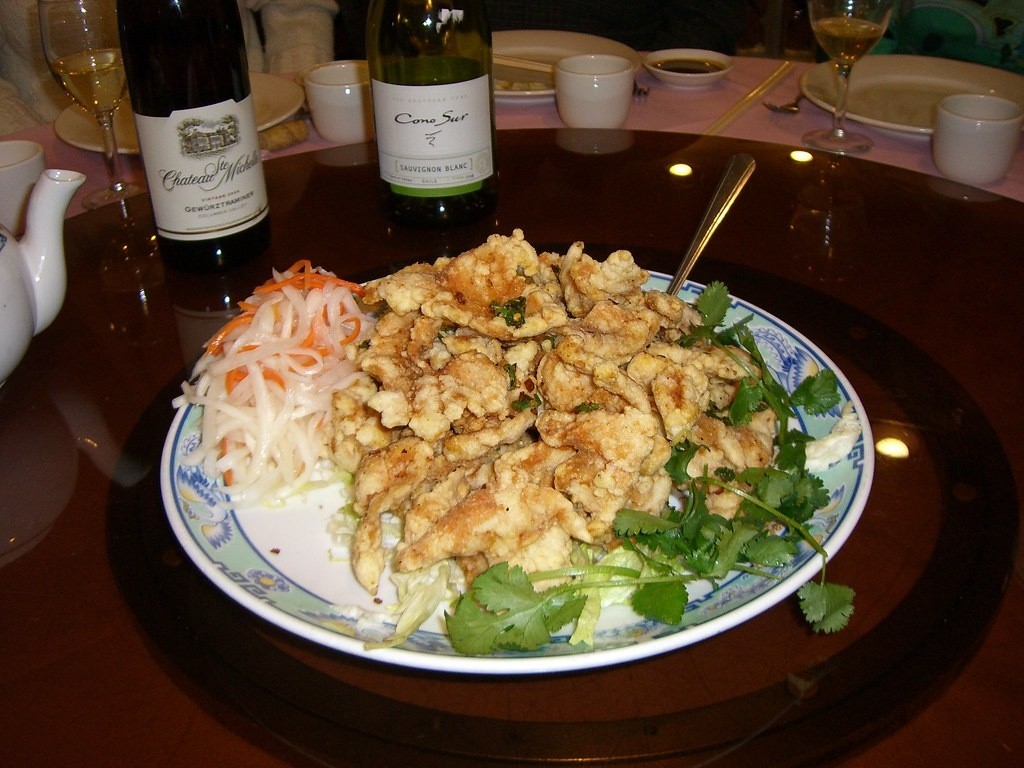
[0,167,86,388]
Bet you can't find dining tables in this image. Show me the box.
[0,54,1024,768]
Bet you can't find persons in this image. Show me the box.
[815,0,1024,78]
[0,0,339,125]
[479,0,750,55]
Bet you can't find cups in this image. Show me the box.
[303,64,374,144]
[932,94,1022,185]
[0,139,47,237]
[556,54,635,129]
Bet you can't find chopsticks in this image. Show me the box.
[702,60,797,135]
[492,53,554,72]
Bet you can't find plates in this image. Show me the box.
[295,60,367,87]
[801,54,1024,134]
[55,72,304,153]
[157,265,871,674]
[488,29,642,103]
[642,49,735,88]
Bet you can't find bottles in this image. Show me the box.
[366,0,500,225]
[116,0,271,267]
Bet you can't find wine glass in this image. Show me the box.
[36,0,148,209]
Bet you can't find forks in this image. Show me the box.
[763,93,805,114]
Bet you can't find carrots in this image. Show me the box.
[203,258,364,487]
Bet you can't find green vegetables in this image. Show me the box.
[443,280,858,653]
